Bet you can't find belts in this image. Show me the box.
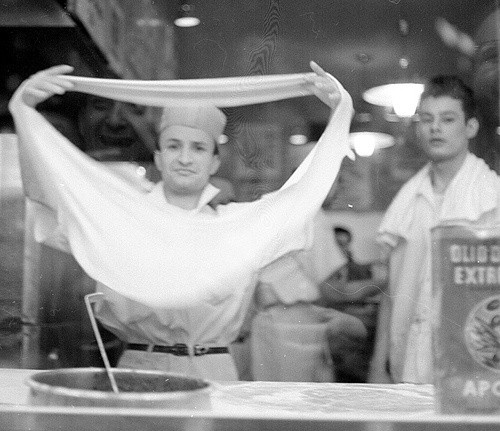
[126,343,229,355]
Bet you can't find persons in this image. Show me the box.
[79,88,151,168]
[8,61,354,383]
[250,141,390,382]
[331,224,352,250]
[366,73,500,382]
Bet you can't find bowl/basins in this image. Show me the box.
[39,321,123,369]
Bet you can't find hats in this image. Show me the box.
[160,100,227,154]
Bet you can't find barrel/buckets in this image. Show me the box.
[26,367,215,412]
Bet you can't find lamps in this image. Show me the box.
[362,19,430,117]
[173,0,201,27]
[350,52,396,156]
